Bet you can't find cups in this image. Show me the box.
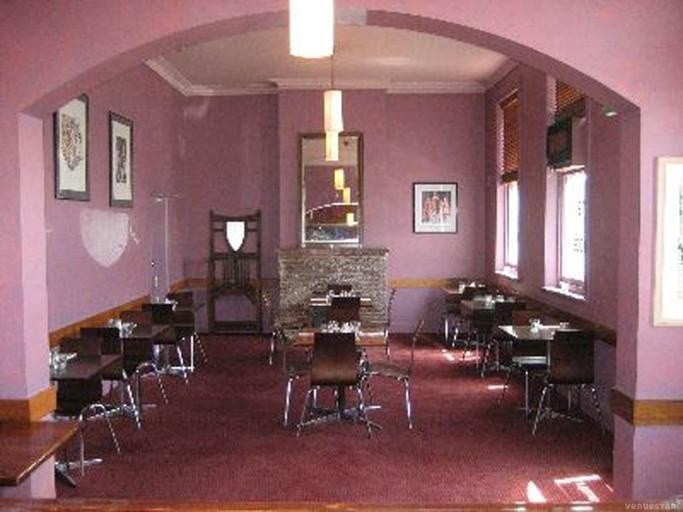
[457,281,477,292]
[105,319,139,333]
[477,291,506,307]
[48,344,72,370]
[527,318,571,336]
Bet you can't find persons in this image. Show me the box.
[424,193,452,225]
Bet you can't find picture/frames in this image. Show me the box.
[649,155,683,329]
[50,94,91,202]
[410,181,459,235]
[107,109,135,210]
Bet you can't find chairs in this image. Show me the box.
[435,274,607,435]
[49,289,210,472]
[253,277,424,441]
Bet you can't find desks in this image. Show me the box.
[0,415,81,485]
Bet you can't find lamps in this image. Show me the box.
[342,187,350,205]
[334,167,343,191]
[323,130,338,162]
[345,212,354,227]
[286,1,333,59]
[322,54,344,134]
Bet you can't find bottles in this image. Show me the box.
[318,318,362,339]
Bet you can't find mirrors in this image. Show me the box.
[298,129,363,248]
[225,219,246,253]
